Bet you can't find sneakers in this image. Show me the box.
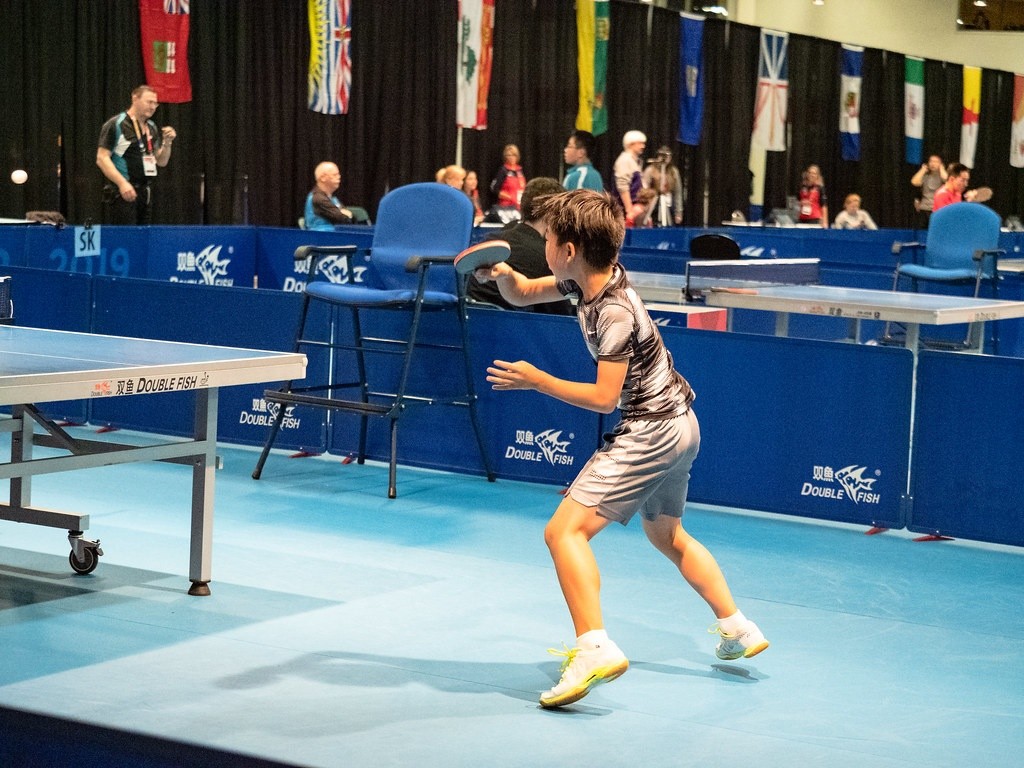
[540,639,630,707]
[707,615,769,659]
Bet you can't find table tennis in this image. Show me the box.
[11,170,28,184]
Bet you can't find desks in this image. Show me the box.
[622,254,1024,354]
[0,325,309,596]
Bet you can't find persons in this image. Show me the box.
[91,85,178,226]
[798,165,875,230]
[910,153,977,230]
[436,132,686,317]
[474,189,771,709]
[305,162,357,228]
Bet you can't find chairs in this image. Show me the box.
[250,183,496,496]
[882,202,1002,350]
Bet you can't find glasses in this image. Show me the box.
[147,99,160,108]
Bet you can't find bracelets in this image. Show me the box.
[161,140,173,147]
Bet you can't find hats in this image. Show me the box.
[622,130,647,149]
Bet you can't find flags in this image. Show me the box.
[139,0,191,103]
[674,12,706,146]
[751,27,789,151]
[573,0,610,137]
[1009,73,1024,168]
[456,0,495,130]
[960,65,981,169]
[305,0,351,117]
[903,54,926,167]
[837,43,864,163]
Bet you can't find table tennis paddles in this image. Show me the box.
[453,239,512,285]
[711,287,759,295]
[963,187,993,203]
